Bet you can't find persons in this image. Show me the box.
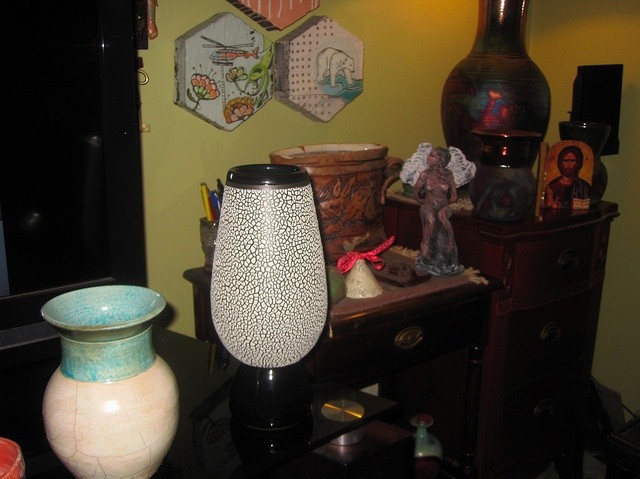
[541,147,591,215]
[414,149,460,262]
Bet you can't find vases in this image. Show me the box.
[39,283,180,479]
[408,414,444,476]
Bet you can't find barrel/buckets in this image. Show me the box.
[268,140,391,268]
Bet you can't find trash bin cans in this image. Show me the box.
[0,437,22,479]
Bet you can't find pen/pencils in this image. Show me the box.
[201,183,213,221]
[217,179,224,207]
[210,189,222,219]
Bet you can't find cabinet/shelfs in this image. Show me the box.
[381,192,621,478]
[183,244,488,476]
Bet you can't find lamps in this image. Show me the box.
[210,165,329,473]
